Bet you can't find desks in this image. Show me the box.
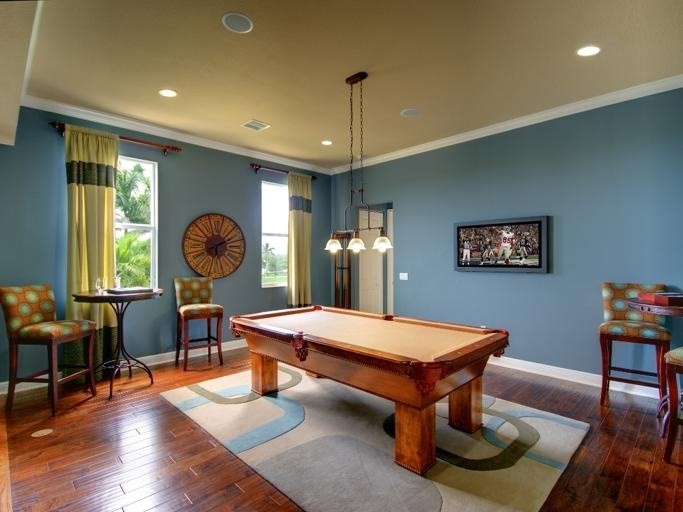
[226,300,511,474]
[625,297,682,438]
[70,284,163,399]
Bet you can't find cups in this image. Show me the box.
[111,277,121,289]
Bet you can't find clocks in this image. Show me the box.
[182,212,245,279]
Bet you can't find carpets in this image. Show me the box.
[158,359,591,511]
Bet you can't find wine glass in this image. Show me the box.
[94,277,108,296]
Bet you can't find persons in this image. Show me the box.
[457,225,539,267]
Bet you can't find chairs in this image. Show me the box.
[171,275,224,370]
[1,281,100,414]
[599,279,671,409]
[662,344,682,463]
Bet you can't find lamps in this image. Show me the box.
[324,69,394,254]
